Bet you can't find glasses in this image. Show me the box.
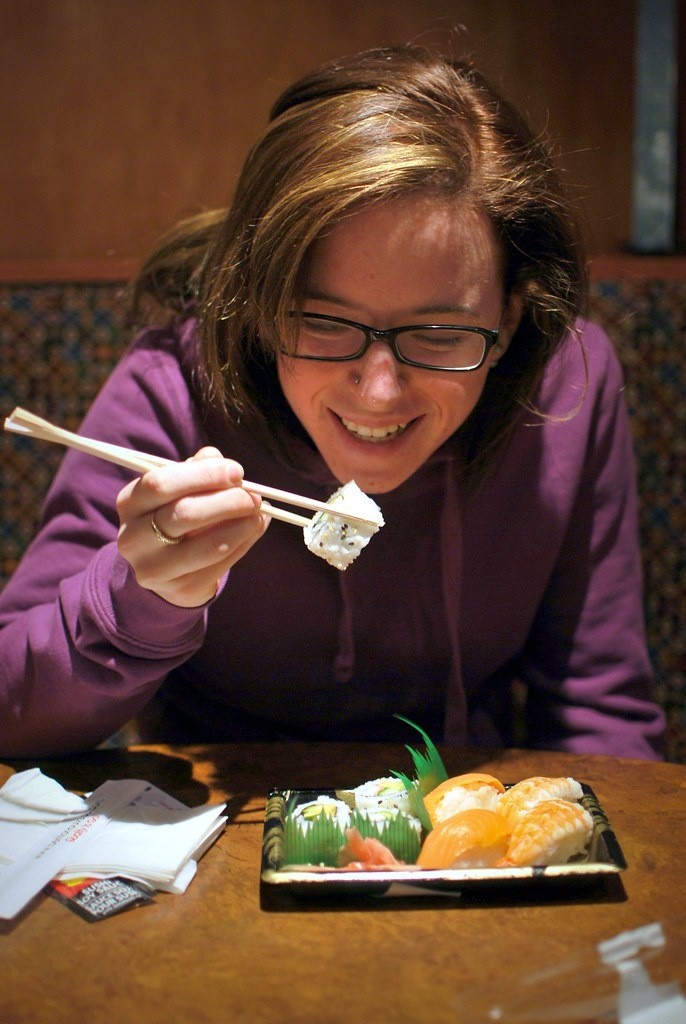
[275,310,506,374]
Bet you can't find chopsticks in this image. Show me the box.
[3,408,378,531]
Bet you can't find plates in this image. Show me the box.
[261,782,627,883]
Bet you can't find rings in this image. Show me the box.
[151,512,188,551]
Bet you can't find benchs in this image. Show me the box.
[1,253,685,756]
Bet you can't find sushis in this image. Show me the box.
[302,478,385,571]
[281,773,595,870]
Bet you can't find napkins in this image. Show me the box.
[53,803,228,894]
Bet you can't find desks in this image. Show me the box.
[0,742,686,1024]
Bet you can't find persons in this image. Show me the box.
[0,47,668,767]
